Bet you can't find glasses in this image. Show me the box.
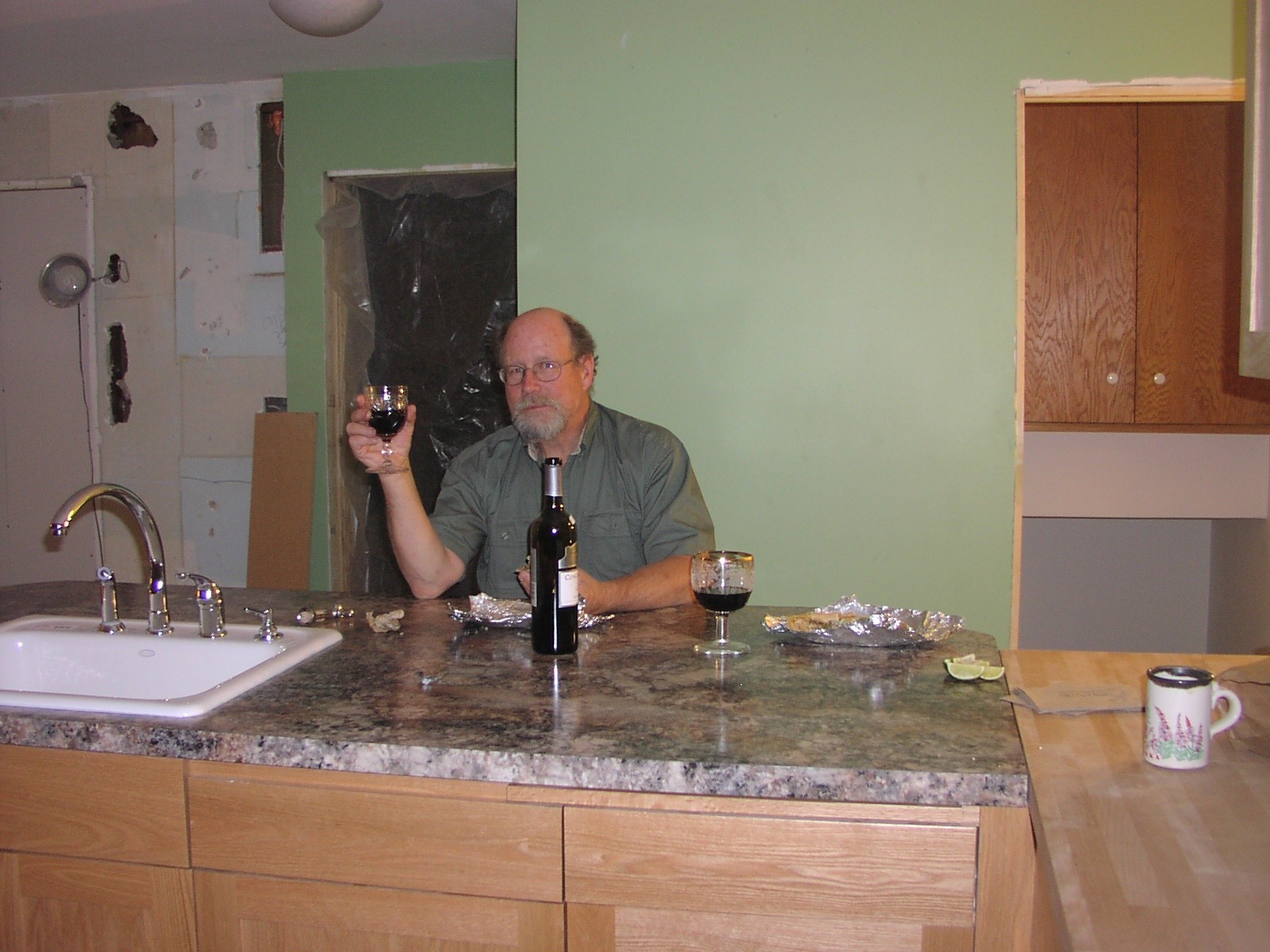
[499,351,586,387]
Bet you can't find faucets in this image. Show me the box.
[96,565,127,635]
[49,482,176,637]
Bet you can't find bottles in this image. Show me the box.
[529,457,579,655]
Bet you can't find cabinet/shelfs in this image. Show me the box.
[1025,102,1269,436]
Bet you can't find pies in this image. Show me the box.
[786,612,840,631]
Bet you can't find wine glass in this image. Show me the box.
[690,550,755,656]
[363,386,410,474]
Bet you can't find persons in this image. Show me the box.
[346,308,714,615]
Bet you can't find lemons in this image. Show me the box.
[943,654,1005,680]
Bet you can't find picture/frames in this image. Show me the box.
[244,97,283,272]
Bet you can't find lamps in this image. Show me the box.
[269,0,384,38]
[38,253,122,311]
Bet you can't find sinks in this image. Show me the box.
[0,612,344,718]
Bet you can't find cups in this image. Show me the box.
[1144,665,1242,770]
